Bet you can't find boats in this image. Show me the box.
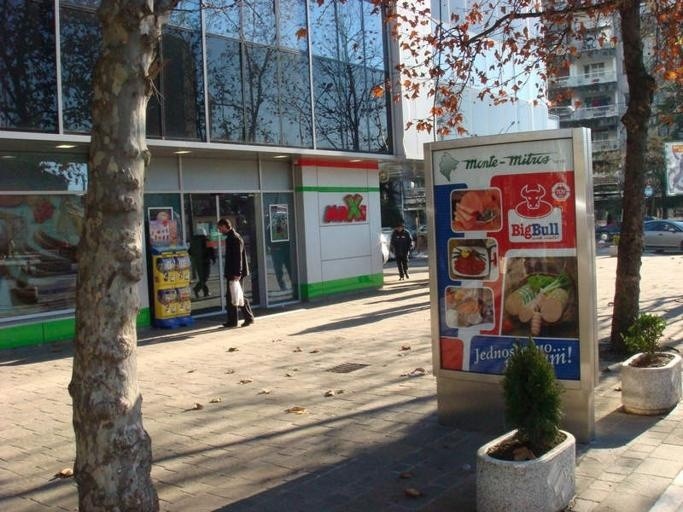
[8,259,78,305]
[33,227,80,262]
[1,238,41,278]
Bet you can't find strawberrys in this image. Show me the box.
[32,199,54,220]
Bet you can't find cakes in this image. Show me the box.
[451,245,487,275]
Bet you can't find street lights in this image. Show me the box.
[398,175,414,224]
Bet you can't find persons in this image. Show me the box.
[268,217,295,292]
[189,225,217,299]
[216,219,256,328]
[389,223,414,281]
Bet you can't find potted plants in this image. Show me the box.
[618,311,681,418]
[475,335,580,510]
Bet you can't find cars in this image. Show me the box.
[380,226,392,235]
[614,219,682,252]
[640,216,658,222]
[596,222,625,241]
[412,225,428,242]
